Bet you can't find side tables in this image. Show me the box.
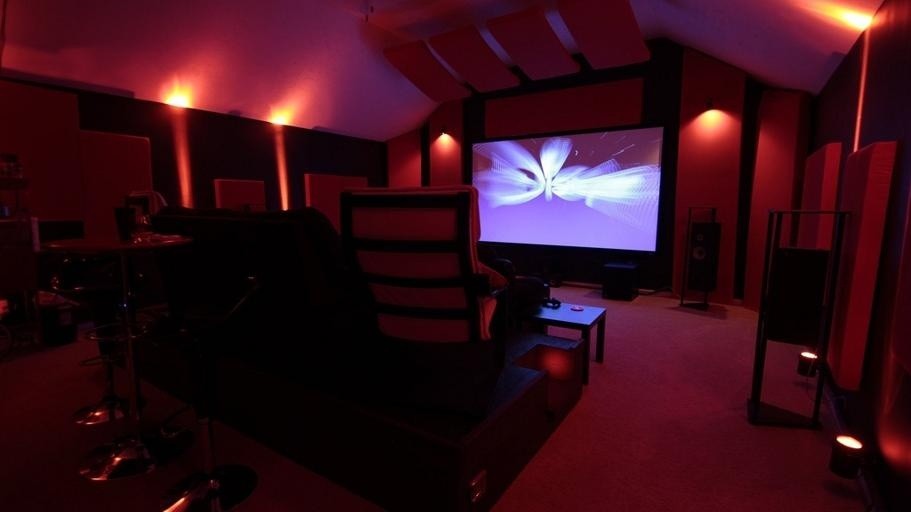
[536,291,607,385]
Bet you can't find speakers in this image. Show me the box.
[688,219,716,292]
[601,263,638,300]
[766,246,833,347]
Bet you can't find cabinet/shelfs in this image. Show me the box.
[1,179,46,350]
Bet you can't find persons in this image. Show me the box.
[477,261,509,289]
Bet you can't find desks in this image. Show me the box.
[39,232,193,479]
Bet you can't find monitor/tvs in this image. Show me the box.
[468,122,676,254]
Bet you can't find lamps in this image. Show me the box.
[831,436,885,482]
[797,350,846,408]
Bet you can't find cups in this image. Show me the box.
[114,207,136,241]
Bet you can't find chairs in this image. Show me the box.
[59,281,145,422]
[341,186,508,417]
[159,277,262,511]
